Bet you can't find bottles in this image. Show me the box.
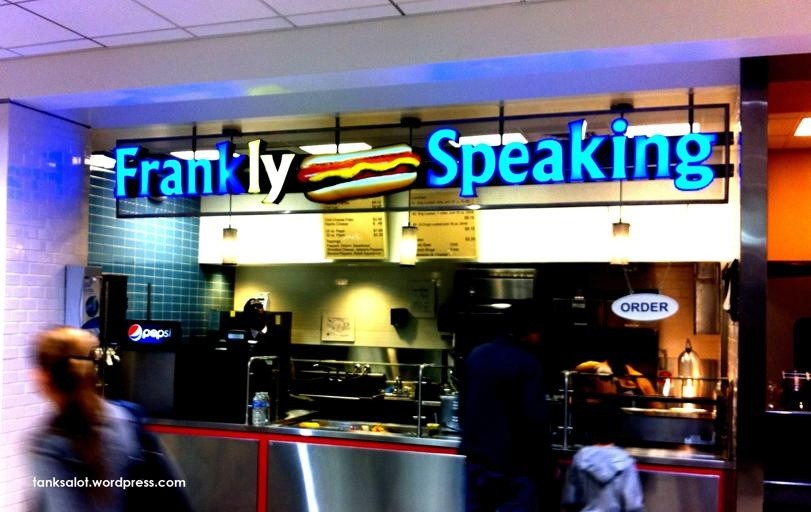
[252,392,271,426]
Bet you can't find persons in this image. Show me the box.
[457,306,561,512]
[561,408,645,511]
[30,326,196,512]
[575,340,666,408]
[240,299,290,356]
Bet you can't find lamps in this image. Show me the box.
[609,100,635,266]
[221,125,243,265]
[677,337,703,378]
[399,117,423,267]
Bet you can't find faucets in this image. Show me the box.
[393,375,403,394]
[312,363,371,383]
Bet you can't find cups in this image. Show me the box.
[426,423,440,437]
